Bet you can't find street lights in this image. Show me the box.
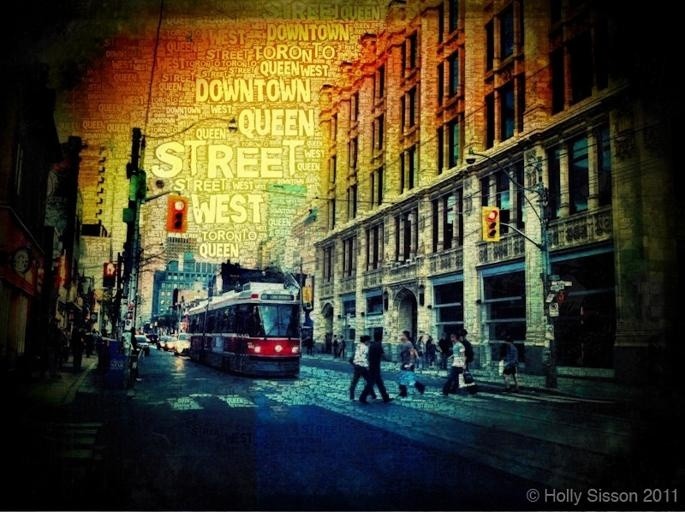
[124,117,238,377]
[465,147,557,387]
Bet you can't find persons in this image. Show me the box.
[338,335,345,358]
[425,336,432,344]
[416,335,426,370]
[442,332,479,397]
[438,333,449,370]
[348,335,378,402]
[502,336,521,392]
[45,315,99,380]
[450,327,475,391]
[331,334,338,358]
[395,330,426,395]
[360,333,395,405]
[305,336,313,355]
[428,339,441,365]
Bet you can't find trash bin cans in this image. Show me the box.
[106,342,125,393]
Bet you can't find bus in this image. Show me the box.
[189,282,301,379]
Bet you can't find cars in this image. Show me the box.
[134,335,150,356]
[156,333,191,356]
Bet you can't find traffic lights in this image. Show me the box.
[166,195,189,233]
[103,262,116,287]
[481,206,500,242]
